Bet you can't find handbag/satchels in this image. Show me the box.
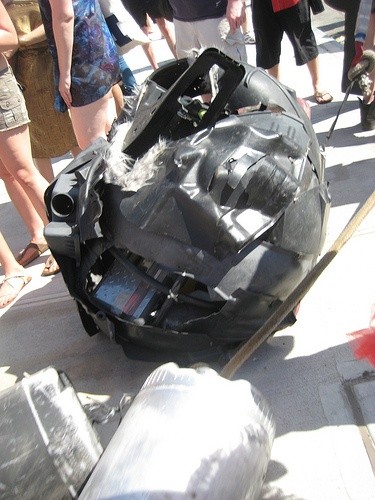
[99,0,151,56]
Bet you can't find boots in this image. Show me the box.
[357,96,375,130]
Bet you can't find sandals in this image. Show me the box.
[0,271,33,309]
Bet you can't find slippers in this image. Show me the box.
[42,257,60,276]
[314,90,333,104]
[15,243,49,267]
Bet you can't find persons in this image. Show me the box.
[249,0,333,113]
[0,233,32,310]
[38,0,128,172]
[124,0,375,134]
[0,0,93,277]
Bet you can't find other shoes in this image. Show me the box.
[243,32,255,43]
